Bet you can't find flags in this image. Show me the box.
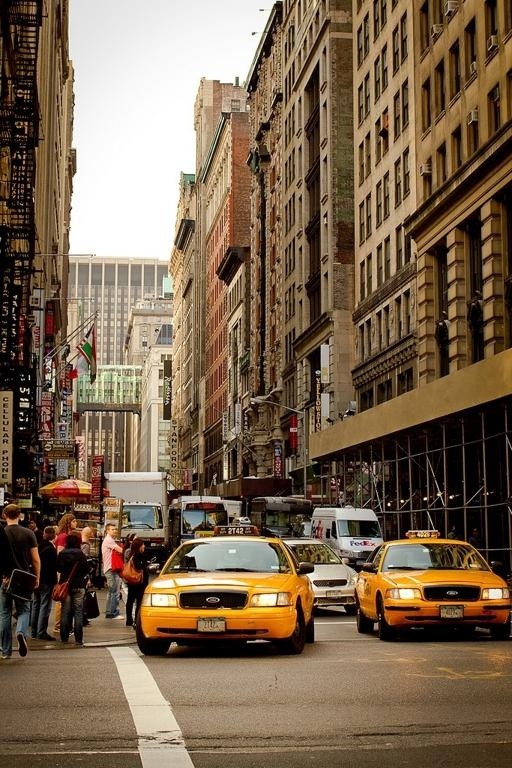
[65,321,99,386]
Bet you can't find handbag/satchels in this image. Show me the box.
[123,569,143,584]
[83,591,100,618]
[6,569,37,600]
[112,544,124,571]
[51,581,70,601]
[123,560,141,583]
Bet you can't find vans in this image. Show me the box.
[310,506,385,567]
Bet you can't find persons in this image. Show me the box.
[125,536,158,626]
[0,513,99,645]
[101,523,125,621]
[0,502,41,660]
[123,532,150,564]
[447,523,486,560]
[311,519,324,538]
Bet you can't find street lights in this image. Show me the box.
[249,397,307,499]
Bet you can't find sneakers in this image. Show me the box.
[32,628,85,646]
[112,615,125,620]
[17,633,27,657]
[1,652,12,658]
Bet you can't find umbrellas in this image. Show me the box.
[38,475,93,510]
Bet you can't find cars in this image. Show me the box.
[276,535,358,615]
[135,526,317,655]
[354,529,511,641]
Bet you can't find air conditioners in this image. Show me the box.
[467,110,478,126]
[486,34,498,51]
[444,1,459,16]
[430,24,444,38]
[419,163,431,176]
[470,61,477,76]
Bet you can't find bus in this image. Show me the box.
[168,495,230,560]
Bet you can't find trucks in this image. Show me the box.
[223,496,313,538]
[103,472,168,561]
